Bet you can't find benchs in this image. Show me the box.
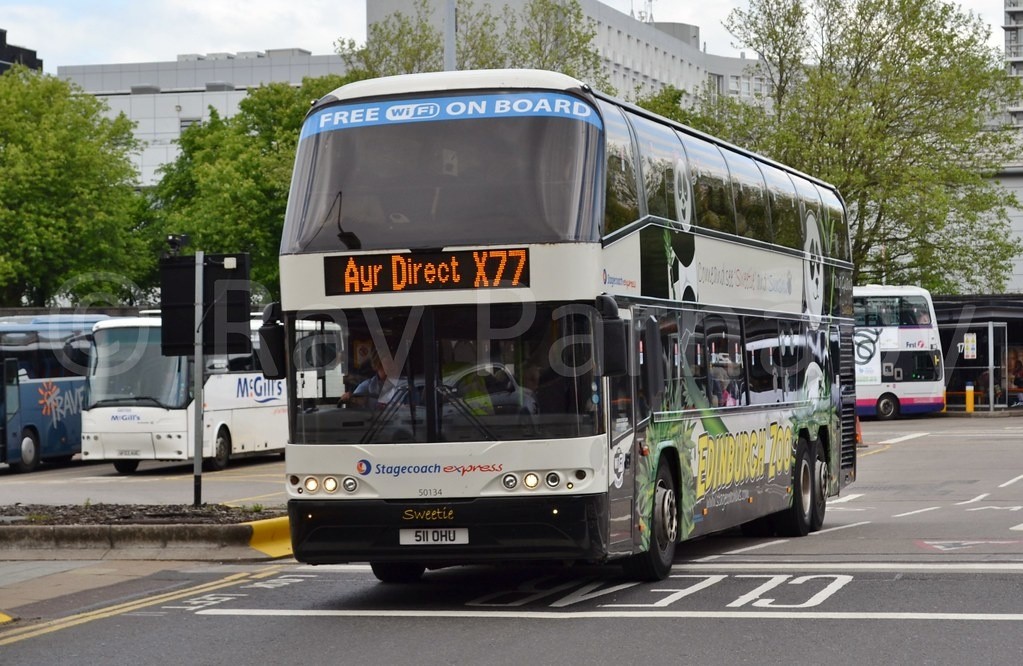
[996,388,1023,405]
[946,391,984,412]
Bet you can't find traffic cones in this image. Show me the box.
[854,414,869,447]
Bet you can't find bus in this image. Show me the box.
[853,283,944,421]
[737,331,824,409]
[255,68,858,597]
[0,314,112,475]
[63,309,344,478]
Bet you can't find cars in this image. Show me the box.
[390,359,539,416]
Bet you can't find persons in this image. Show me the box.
[349,346,416,409]
[711,367,737,407]
[979,362,1002,405]
[1011,359,1023,408]
